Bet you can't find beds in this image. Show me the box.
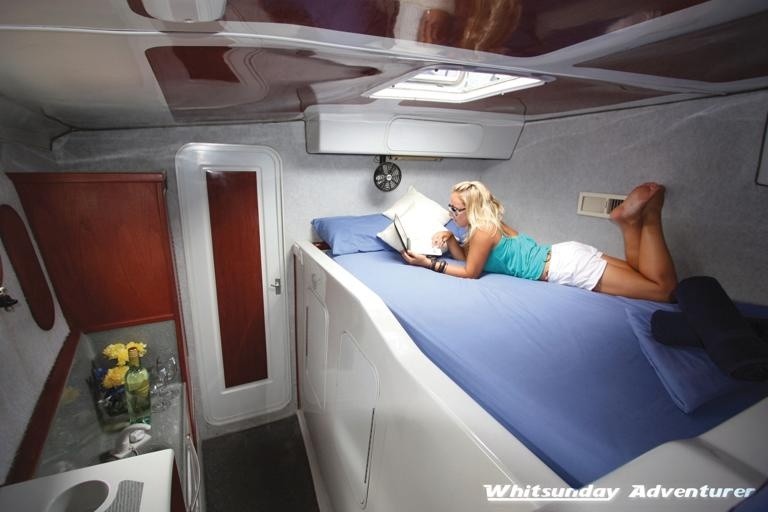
[297,235,765,512]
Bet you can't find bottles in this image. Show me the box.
[124,347,151,429]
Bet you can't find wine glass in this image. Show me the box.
[156,352,180,406]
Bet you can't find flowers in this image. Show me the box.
[98,339,151,414]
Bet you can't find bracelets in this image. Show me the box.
[428,257,437,270]
[438,260,446,272]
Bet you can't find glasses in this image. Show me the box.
[448,204,466,213]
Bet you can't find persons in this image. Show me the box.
[401,181,678,303]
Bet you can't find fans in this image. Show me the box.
[372,154,401,193]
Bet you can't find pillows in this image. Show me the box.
[311,188,467,256]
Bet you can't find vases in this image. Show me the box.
[90,375,153,434]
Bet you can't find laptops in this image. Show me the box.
[394,214,443,258]
